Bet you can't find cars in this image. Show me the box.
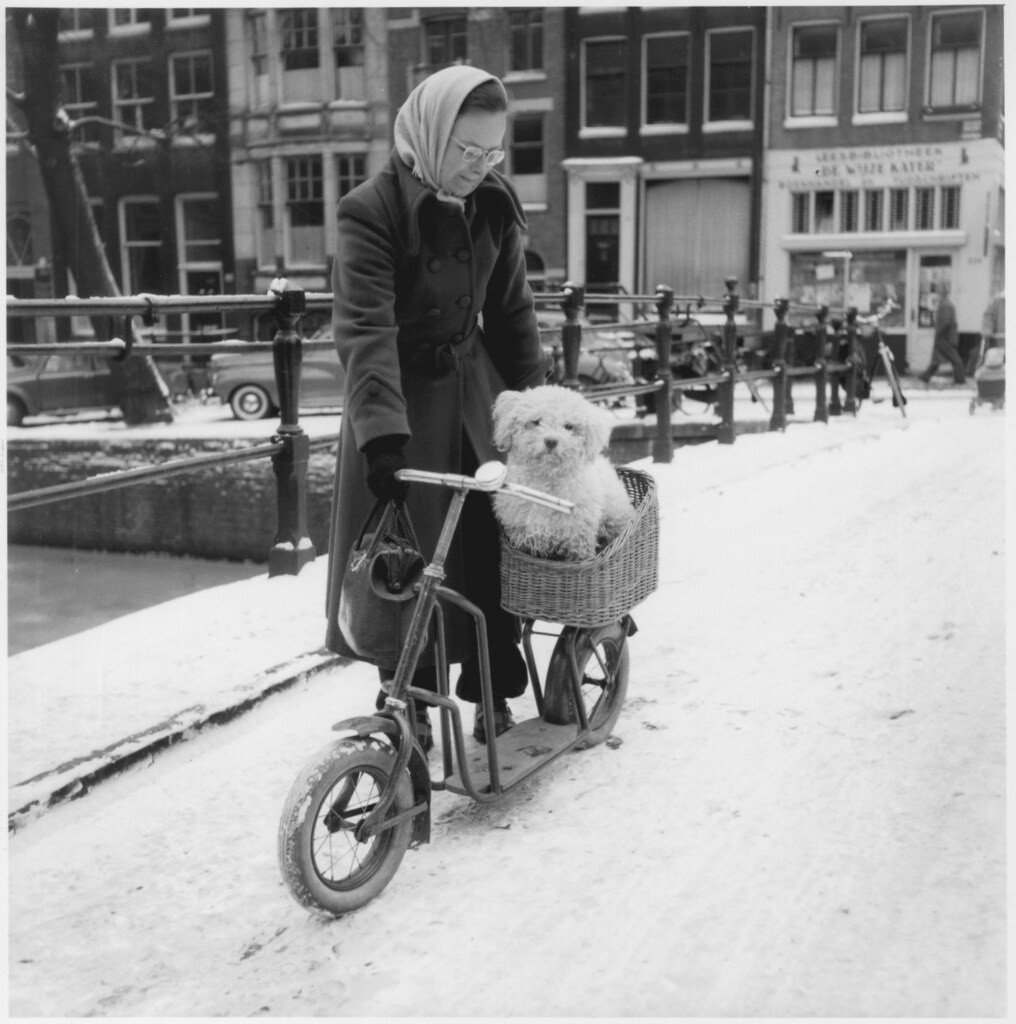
[7,333,120,429]
[208,299,728,423]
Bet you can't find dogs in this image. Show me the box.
[492,385,636,561]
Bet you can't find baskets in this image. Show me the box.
[497,465,659,627]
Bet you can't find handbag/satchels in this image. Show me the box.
[336,490,435,660]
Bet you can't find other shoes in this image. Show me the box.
[413,705,432,749]
[473,699,518,747]
[954,379,965,384]
[918,374,929,383]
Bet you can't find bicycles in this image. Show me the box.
[857,296,912,420]
[273,456,636,919]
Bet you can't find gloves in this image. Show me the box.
[364,442,409,509]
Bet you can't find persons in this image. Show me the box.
[323,65,545,761]
[918,279,966,385]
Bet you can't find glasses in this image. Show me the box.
[451,134,506,166]
